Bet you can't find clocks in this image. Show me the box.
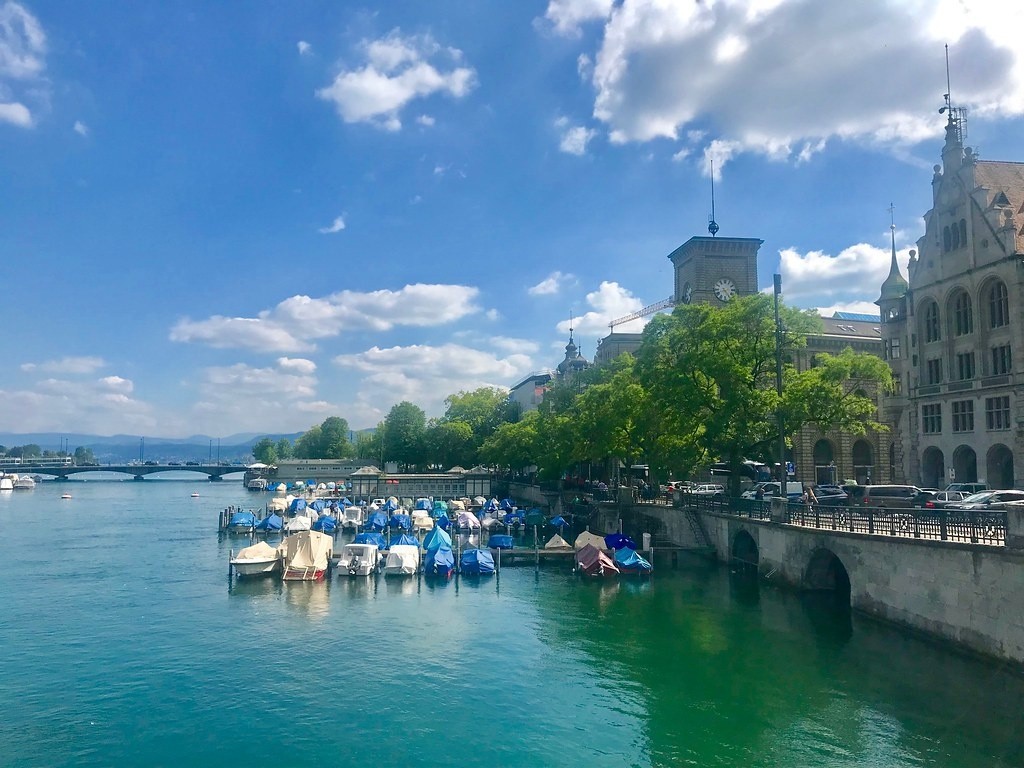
[714,278,736,301]
[682,282,692,304]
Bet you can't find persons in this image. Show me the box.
[771,476,777,482]
[598,481,608,497]
[755,486,765,500]
[801,487,818,516]
[637,479,649,498]
[565,473,590,491]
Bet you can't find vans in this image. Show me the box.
[740,481,804,504]
[848,484,925,519]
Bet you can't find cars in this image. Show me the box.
[683,483,726,497]
[944,482,991,494]
[909,491,973,522]
[944,490,1024,524]
[145,461,199,465]
[789,489,848,510]
[661,482,695,495]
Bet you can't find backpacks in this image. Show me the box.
[808,495,814,502]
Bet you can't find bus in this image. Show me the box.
[0,457,73,468]
[709,460,771,486]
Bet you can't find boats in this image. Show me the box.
[459,546,494,574]
[62,494,72,498]
[13,475,36,489]
[611,545,654,575]
[230,478,638,550]
[426,542,456,578]
[0,478,13,490]
[191,492,200,497]
[574,542,620,578]
[382,544,422,577]
[336,544,385,577]
[228,540,282,576]
[282,530,335,579]
